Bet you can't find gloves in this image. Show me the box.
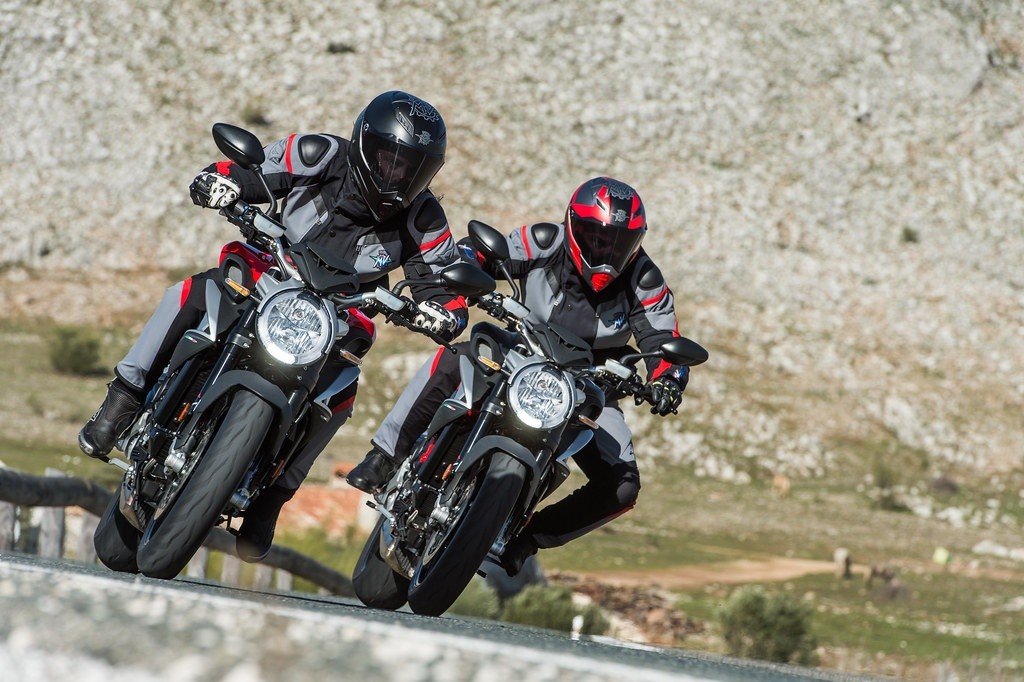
[634,376,682,416]
[411,300,458,345]
[197,173,243,209]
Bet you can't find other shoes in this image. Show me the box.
[236,485,294,563]
[81,376,143,458]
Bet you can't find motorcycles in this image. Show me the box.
[338,220,713,613]
[83,124,499,584]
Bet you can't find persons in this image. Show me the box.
[79,90,468,563]
[347,177,690,578]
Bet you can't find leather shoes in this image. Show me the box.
[348,448,398,494]
[500,530,539,578]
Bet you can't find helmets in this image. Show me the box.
[564,176,648,293]
[349,91,447,223]
